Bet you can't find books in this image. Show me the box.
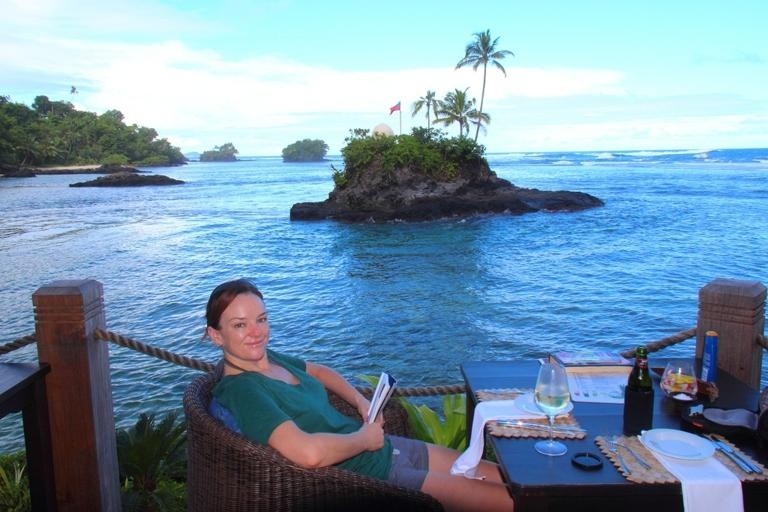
[368,370,395,423]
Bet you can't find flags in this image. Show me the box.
[391,102,400,115]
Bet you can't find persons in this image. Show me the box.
[200,278,515,512]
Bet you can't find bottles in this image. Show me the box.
[622,342,654,436]
[701,329,720,384]
[660,361,699,400]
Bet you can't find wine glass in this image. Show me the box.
[535,363,571,456]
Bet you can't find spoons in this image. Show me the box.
[606,432,652,471]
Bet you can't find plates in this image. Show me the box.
[670,392,697,406]
[641,428,715,460]
[514,394,574,414]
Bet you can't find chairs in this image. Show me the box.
[182,371,444,512]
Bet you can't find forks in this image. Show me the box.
[605,439,632,475]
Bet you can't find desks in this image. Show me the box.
[0,361,59,512]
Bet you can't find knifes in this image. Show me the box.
[495,419,590,435]
[701,431,763,476]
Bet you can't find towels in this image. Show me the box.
[637,435,744,512]
[703,407,759,431]
[450,399,570,479]
